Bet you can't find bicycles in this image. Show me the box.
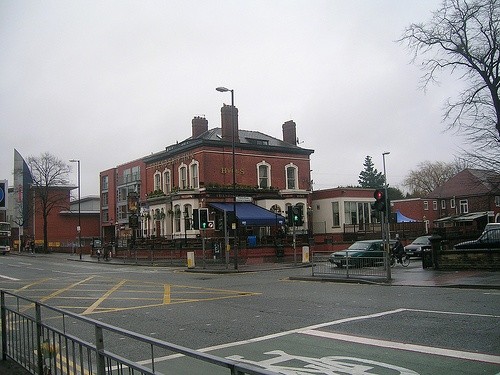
[389,253,410,267]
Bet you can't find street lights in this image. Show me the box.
[70,159,83,259]
[381,151,391,249]
[216,86,238,270]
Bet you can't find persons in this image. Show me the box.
[390,237,406,268]
[25,238,31,254]
[29,239,35,254]
[95,248,102,261]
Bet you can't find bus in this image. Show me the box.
[0,222,10,253]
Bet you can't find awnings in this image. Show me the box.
[206,200,286,228]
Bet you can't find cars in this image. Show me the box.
[330,238,400,270]
[455,227,500,248]
[404,235,433,259]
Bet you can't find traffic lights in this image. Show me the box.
[286,205,294,228]
[192,208,198,230]
[374,187,386,212]
[199,209,208,230]
[294,205,301,227]
